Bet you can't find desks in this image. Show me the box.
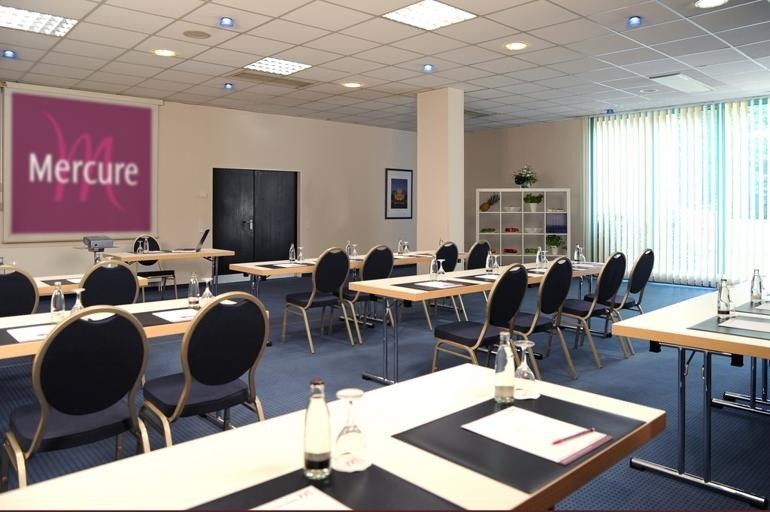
[612,285,770,510]
[0,364,666,510]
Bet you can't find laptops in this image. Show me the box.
[172,229,210,253]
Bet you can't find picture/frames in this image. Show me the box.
[385,168,413,220]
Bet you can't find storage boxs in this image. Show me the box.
[546,214,567,233]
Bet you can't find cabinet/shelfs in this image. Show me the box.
[475,187,571,265]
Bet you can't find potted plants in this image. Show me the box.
[511,160,538,188]
[546,235,568,255]
[523,193,543,212]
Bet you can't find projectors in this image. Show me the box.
[83,236,113,252]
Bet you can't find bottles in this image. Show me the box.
[750,269,762,303]
[50,282,66,317]
[536,246,543,270]
[303,377,331,480]
[187,271,201,307]
[485,251,494,273]
[397,239,404,255]
[289,243,295,264]
[50,237,585,320]
[574,243,580,263]
[493,331,515,405]
[345,240,352,256]
[439,237,443,247]
[716,279,730,318]
[429,255,438,280]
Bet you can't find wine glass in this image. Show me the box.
[541,251,550,269]
[492,255,502,275]
[200,278,214,306]
[728,287,735,317]
[351,243,358,256]
[403,242,410,255]
[331,387,376,472]
[436,259,447,280]
[578,247,585,264]
[761,276,768,301]
[512,341,536,399]
[296,247,305,261]
[68,288,87,313]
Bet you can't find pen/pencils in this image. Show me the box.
[553,427,595,445]
